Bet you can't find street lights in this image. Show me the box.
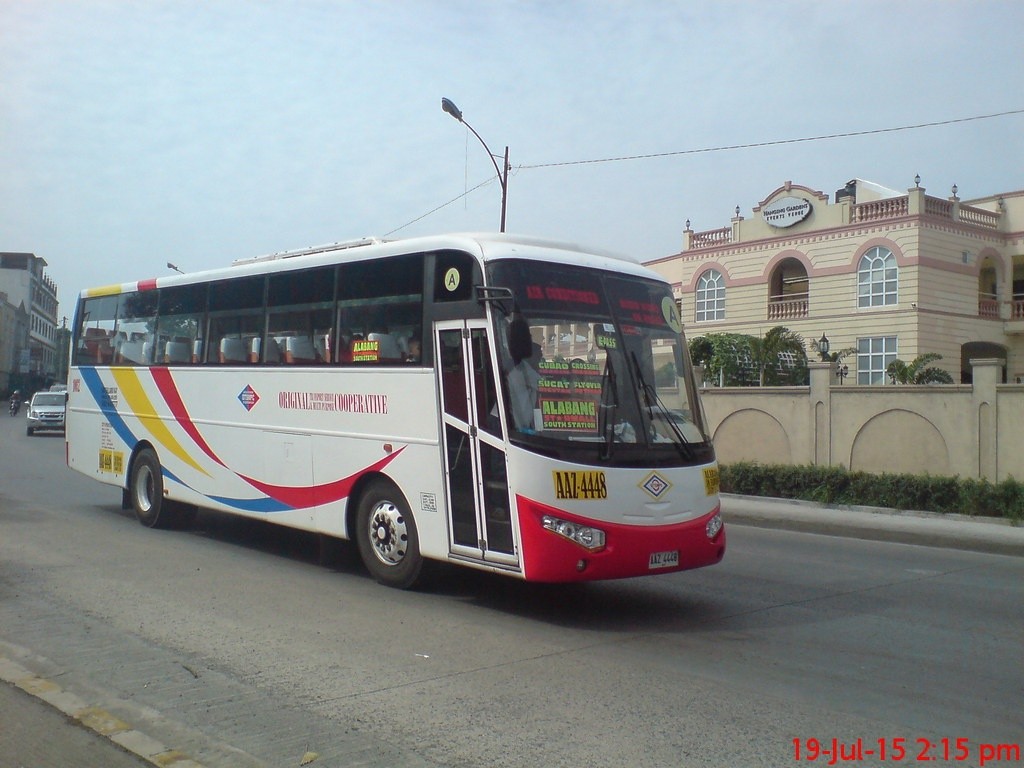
[440,96,508,235]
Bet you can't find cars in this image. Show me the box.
[49,385,67,393]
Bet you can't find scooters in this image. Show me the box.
[9,399,20,416]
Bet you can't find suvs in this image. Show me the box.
[24,391,66,436]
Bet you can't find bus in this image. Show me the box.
[63,233,725,590]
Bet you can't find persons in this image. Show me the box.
[9,390,21,411]
[406,336,421,363]
[343,335,364,360]
[490,343,543,521]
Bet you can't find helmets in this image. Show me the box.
[14,390,18,394]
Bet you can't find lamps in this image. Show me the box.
[998,197,1003,208]
[735,204,740,217]
[914,174,920,187]
[951,184,958,197]
[818,331,829,361]
[686,219,690,230]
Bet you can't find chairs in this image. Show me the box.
[84,328,411,366]
